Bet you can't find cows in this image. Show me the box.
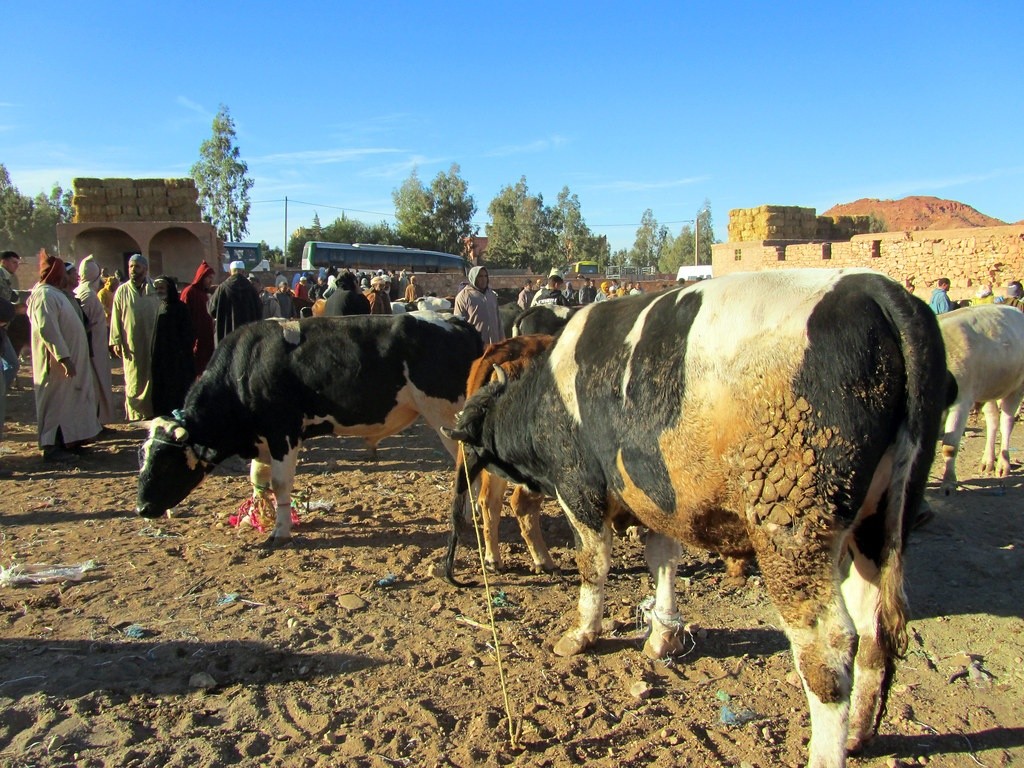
[445,333,557,587]
[126,310,484,546]
[498,300,575,338]
[935,304,1024,496]
[440,267,947,768]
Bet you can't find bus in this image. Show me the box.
[569,260,600,277]
[220,241,267,273]
[605,266,637,281]
[303,241,475,274]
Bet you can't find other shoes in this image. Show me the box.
[100,427,117,434]
[44,442,79,464]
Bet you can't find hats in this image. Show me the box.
[130,254,147,264]
[601,282,608,290]
[230,261,245,270]
[609,286,616,291]
[381,275,391,283]
[370,275,385,285]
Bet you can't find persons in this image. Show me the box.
[561,278,643,305]
[1008,281,1024,303]
[928,278,963,316]
[660,277,703,291]
[517,275,571,310]
[454,266,507,351]
[248,266,437,319]
[0,248,263,468]
